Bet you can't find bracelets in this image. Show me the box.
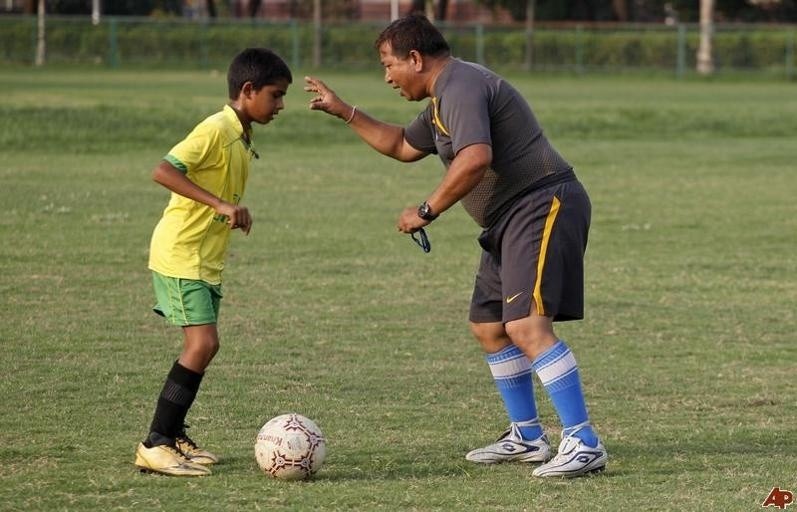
[344,106,356,125]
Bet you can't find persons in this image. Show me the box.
[134,48,293,476]
[304,13,608,479]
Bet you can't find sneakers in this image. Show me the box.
[463,425,554,464]
[529,432,610,480]
[174,432,220,465]
[132,440,214,477]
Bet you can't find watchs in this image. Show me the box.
[417,201,439,221]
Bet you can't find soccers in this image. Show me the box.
[254,414,326,481]
